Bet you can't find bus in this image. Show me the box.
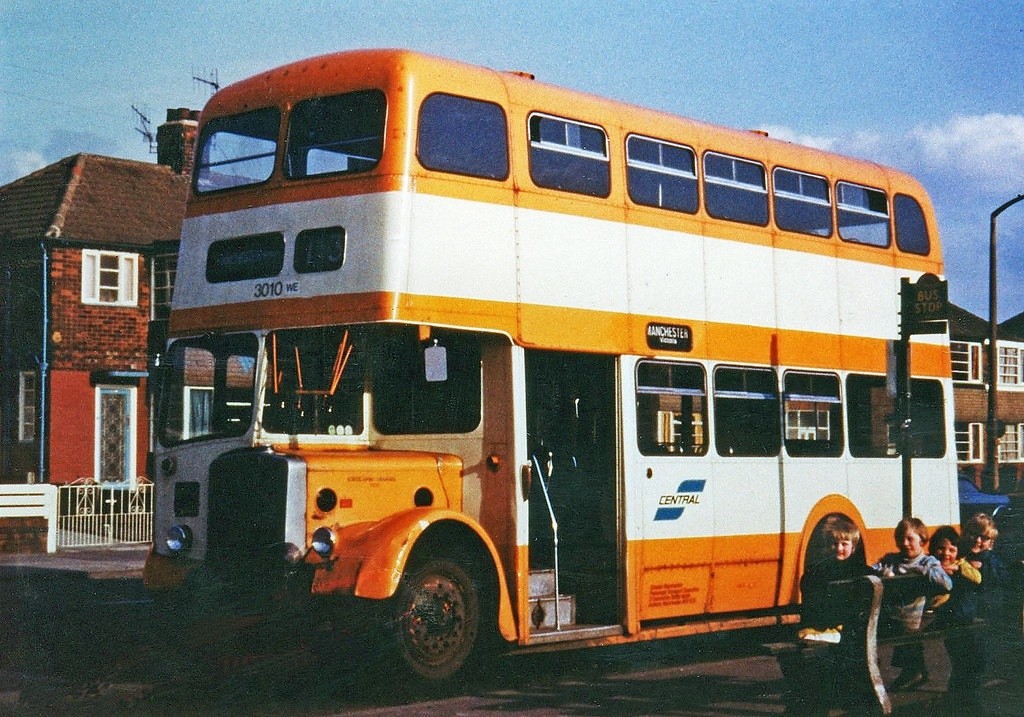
[140,48,965,700]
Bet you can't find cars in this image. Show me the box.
[956,473,1013,522]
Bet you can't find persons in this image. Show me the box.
[800,514,878,647]
[871,514,1013,693]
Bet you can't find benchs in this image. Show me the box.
[759,560,1024,717]
